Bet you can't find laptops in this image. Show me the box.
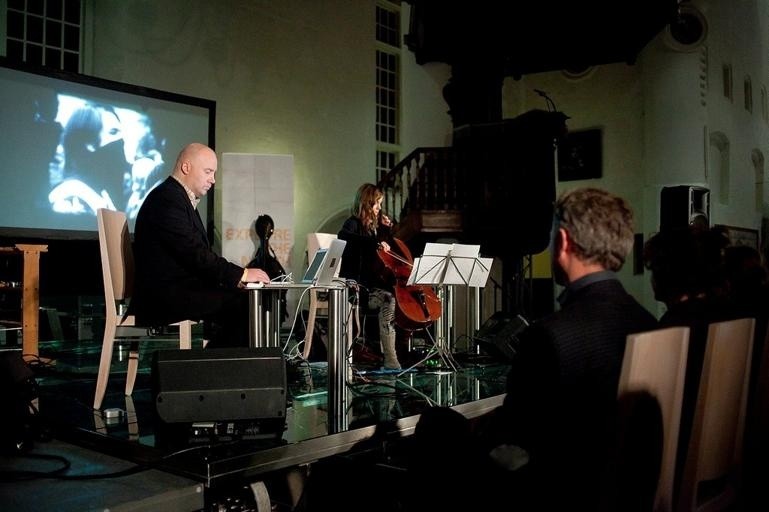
[265,238,347,288]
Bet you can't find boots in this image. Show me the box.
[381,333,403,371]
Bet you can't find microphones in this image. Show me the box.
[534,89,547,97]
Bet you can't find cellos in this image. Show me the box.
[375,212,443,331]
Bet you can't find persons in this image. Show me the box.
[332,181,403,372]
[49,101,131,212]
[128,140,270,352]
[416,184,668,469]
[641,215,769,512]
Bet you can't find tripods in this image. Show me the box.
[395,286,476,378]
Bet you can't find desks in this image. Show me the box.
[245,281,352,379]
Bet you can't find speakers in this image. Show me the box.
[150,346,288,424]
[655,185,710,302]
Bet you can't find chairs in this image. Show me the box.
[616,324,690,511]
[92,208,195,411]
[695,315,761,506]
[302,228,358,364]
